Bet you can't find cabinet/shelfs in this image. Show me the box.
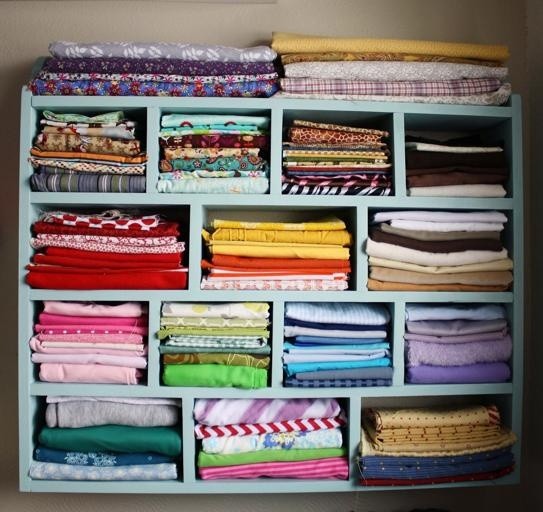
[16,92,524,495]
